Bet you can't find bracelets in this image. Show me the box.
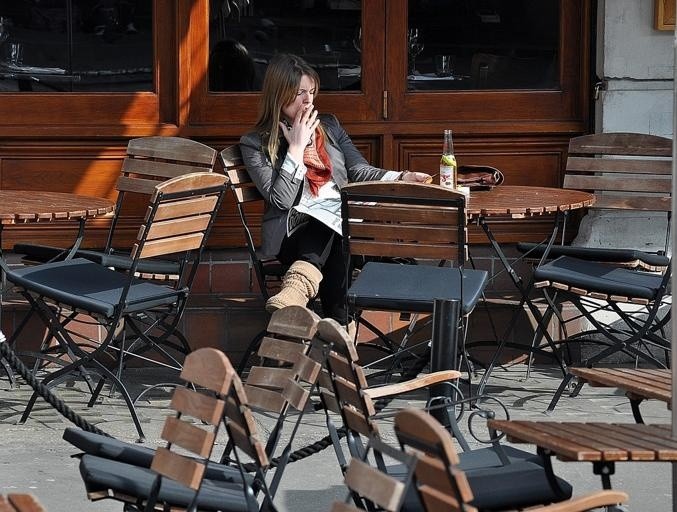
[398,169,411,181]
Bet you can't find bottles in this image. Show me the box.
[440,130,457,191]
[479,64,488,81]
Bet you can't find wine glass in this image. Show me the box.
[322,26,424,77]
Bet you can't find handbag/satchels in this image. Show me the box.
[457,165,505,191]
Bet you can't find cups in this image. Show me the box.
[7,43,23,66]
[433,55,457,76]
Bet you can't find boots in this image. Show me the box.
[266,260,323,314]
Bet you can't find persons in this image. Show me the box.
[241,51,434,343]
[245,9,279,61]
